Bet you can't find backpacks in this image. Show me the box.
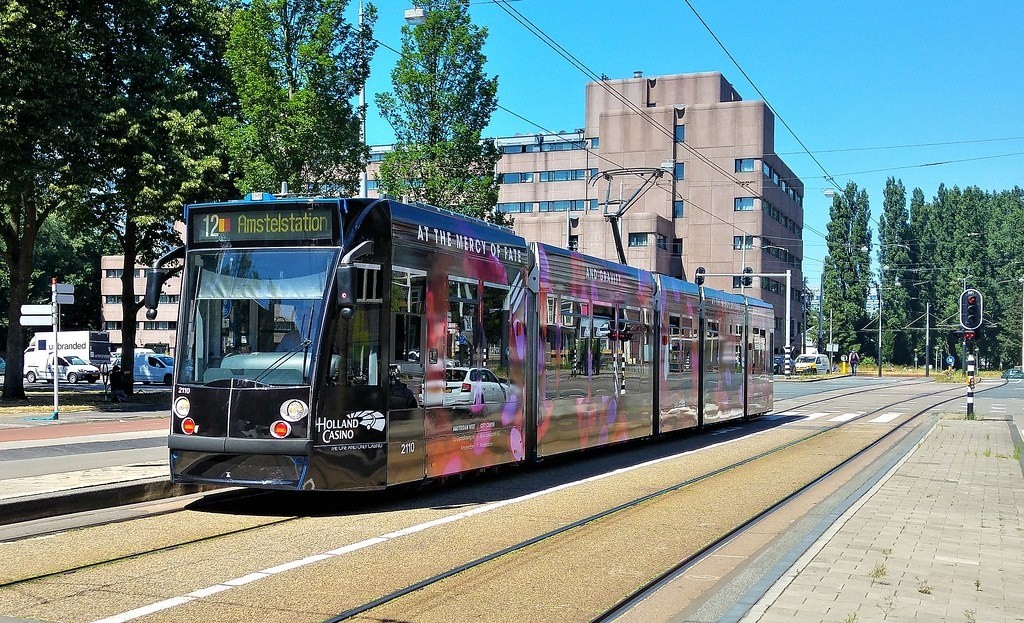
[851,352,857,361]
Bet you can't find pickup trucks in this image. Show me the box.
[22,351,101,385]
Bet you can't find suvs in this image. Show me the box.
[792,354,831,376]
[133,353,175,387]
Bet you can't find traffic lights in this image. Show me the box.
[959,288,983,330]
[964,331,975,340]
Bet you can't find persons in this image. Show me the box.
[849,349,859,376]
[274,305,340,355]
[109,365,131,402]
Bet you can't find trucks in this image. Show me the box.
[24,331,111,372]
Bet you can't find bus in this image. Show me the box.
[167,179,776,496]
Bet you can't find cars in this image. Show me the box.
[0,358,7,390]
[1001,366,1024,379]
[751,355,796,376]
